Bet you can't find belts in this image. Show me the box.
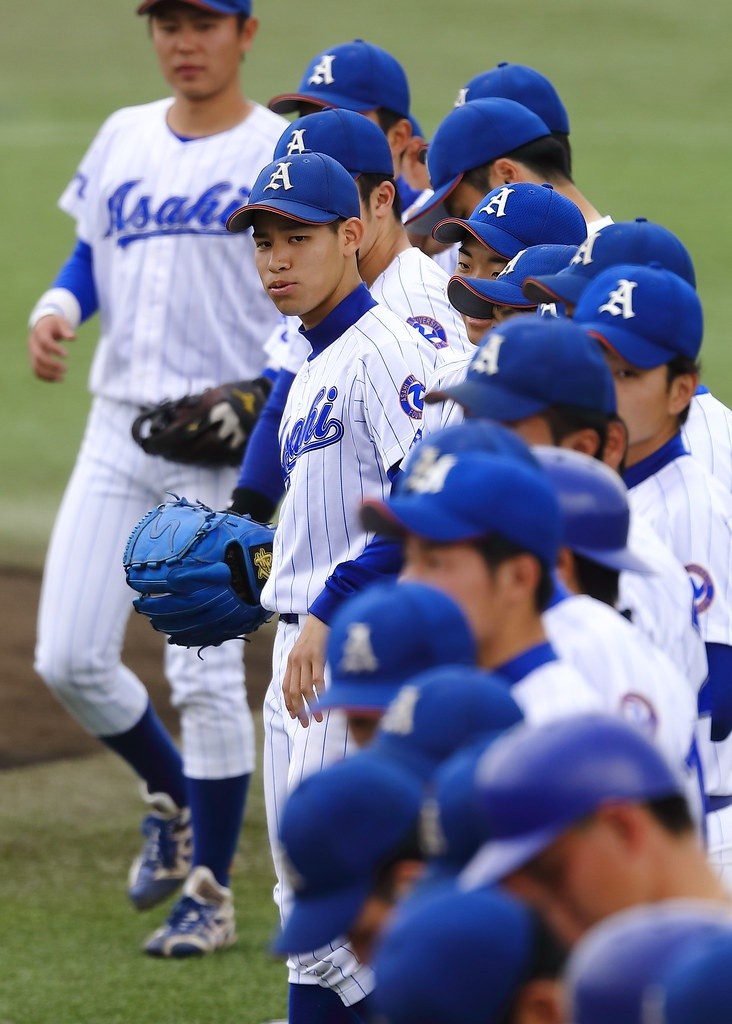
[279,613,299,624]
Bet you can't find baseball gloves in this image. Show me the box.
[122,502,278,649]
[131,376,272,470]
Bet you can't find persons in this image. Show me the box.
[27,0,291,957]
[227,40,732,1024]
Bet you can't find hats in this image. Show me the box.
[572,264,704,368]
[454,63,570,137]
[268,39,410,119]
[432,182,588,261]
[423,316,618,425]
[304,584,477,720]
[276,668,732,1024]
[403,97,552,227]
[447,243,582,319]
[273,107,395,181]
[137,0,252,18]
[520,218,696,316]
[407,115,424,139]
[359,454,562,572]
[226,149,360,233]
[403,420,543,497]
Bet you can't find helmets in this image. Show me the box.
[528,444,656,579]
[452,711,685,893]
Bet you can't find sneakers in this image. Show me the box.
[142,865,237,958]
[128,782,195,910]
